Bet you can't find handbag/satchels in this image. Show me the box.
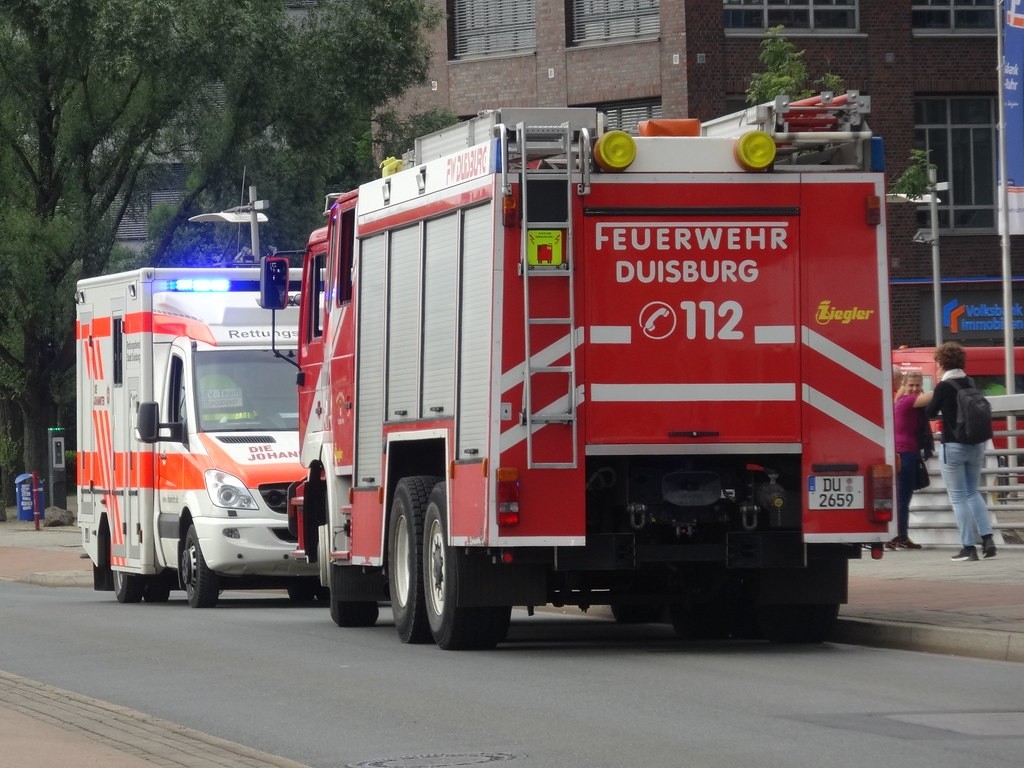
[912,453,930,489]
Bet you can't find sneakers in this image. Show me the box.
[951,546,978,561]
[884,537,896,551]
[981,538,996,559]
[897,538,922,549]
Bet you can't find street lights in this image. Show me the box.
[188,200,270,263]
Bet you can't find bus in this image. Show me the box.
[892,345,1024,497]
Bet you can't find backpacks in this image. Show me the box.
[941,377,993,444]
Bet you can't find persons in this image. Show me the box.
[882,364,936,550]
[926,339,998,562]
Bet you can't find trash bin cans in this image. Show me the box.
[14,472,45,520]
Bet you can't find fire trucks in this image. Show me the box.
[74,262,353,608]
[259,90,898,651]
